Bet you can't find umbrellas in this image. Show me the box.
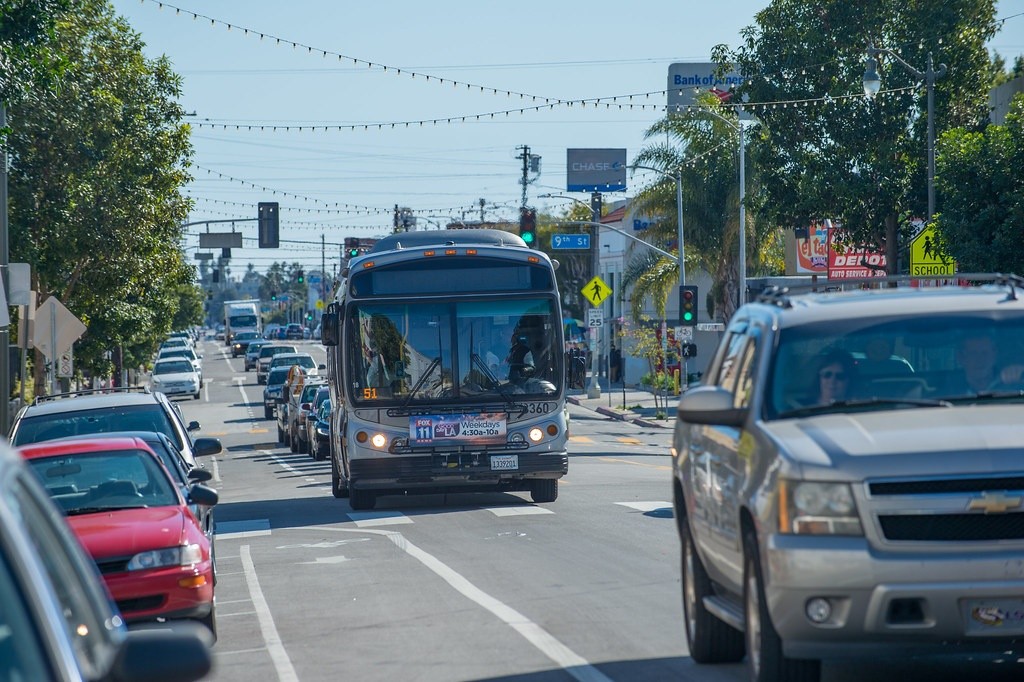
[564,316,586,339]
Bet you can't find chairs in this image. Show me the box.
[276,373,288,384]
[278,361,285,366]
[781,351,862,413]
[91,480,145,505]
[129,420,157,432]
[51,492,88,511]
[48,484,79,497]
[309,389,317,402]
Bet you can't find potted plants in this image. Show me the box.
[640,371,680,396]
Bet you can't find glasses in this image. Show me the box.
[819,371,846,381]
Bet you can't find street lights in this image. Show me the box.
[863,43,947,276]
[612,162,688,396]
[537,194,601,400]
[687,106,746,307]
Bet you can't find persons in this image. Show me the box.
[487,325,536,378]
[367,342,396,386]
[610,345,621,384]
[790,350,856,409]
[858,334,912,374]
[950,330,1024,395]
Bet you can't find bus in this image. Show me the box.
[321,230,568,527]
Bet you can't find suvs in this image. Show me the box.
[670,273,1024,681]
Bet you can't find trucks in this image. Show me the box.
[224,299,264,345]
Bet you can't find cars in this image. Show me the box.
[263,322,320,341]
[150,324,225,401]
[0,385,223,682]
[230,331,330,460]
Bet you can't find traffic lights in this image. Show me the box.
[271,290,276,301]
[680,285,698,327]
[682,344,695,359]
[344,237,359,261]
[308,311,312,321]
[298,270,304,283]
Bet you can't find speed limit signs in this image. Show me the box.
[588,309,603,327]
